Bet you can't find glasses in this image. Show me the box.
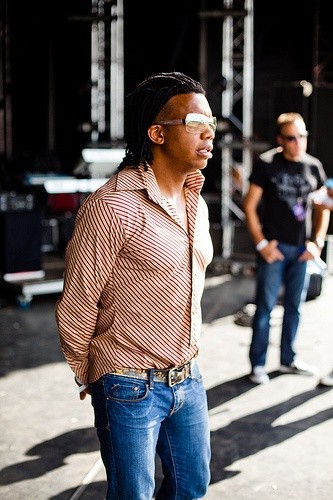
[158,112,217,134]
[280,131,309,142]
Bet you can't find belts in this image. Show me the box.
[109,350,199,387]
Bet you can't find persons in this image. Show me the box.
[56,72,215,500]
[245,113,333,385]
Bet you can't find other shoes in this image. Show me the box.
[279,358,313,376]
[250,365,271,386]
[319,368,333,388]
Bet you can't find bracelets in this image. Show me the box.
[316,238,324,248]
[75,376,88,393]
[256,239,267,251]
